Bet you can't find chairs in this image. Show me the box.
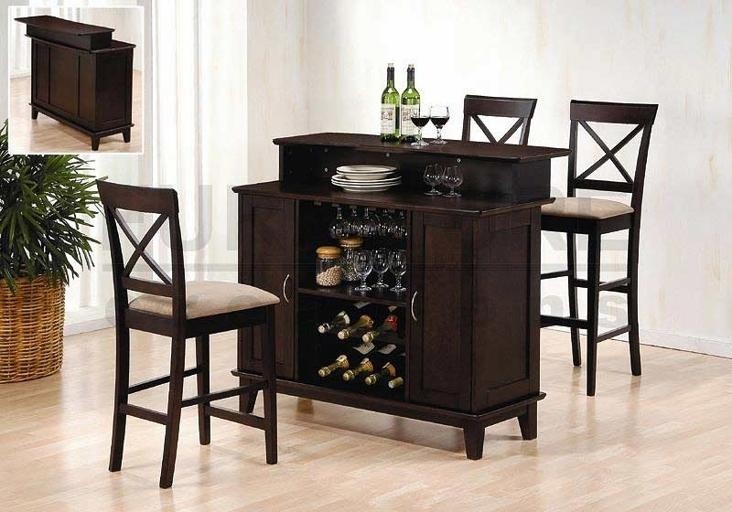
[96,179,282,489]
[541,100,658,396]
[462,94,537,147]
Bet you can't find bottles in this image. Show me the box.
[380,63,422,146]
[315,246,341,287]
[315,303,403,390]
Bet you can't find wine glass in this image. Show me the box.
[423,163,464,198]
[329,204,409,293]
[410,105,451,147]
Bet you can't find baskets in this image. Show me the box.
[0,271,65,384]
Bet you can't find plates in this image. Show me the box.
[331,164,403,194]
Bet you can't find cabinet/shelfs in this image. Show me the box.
[232,132,571,461]
[14,14,136,150]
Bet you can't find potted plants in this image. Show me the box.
[0,117,108,381]
